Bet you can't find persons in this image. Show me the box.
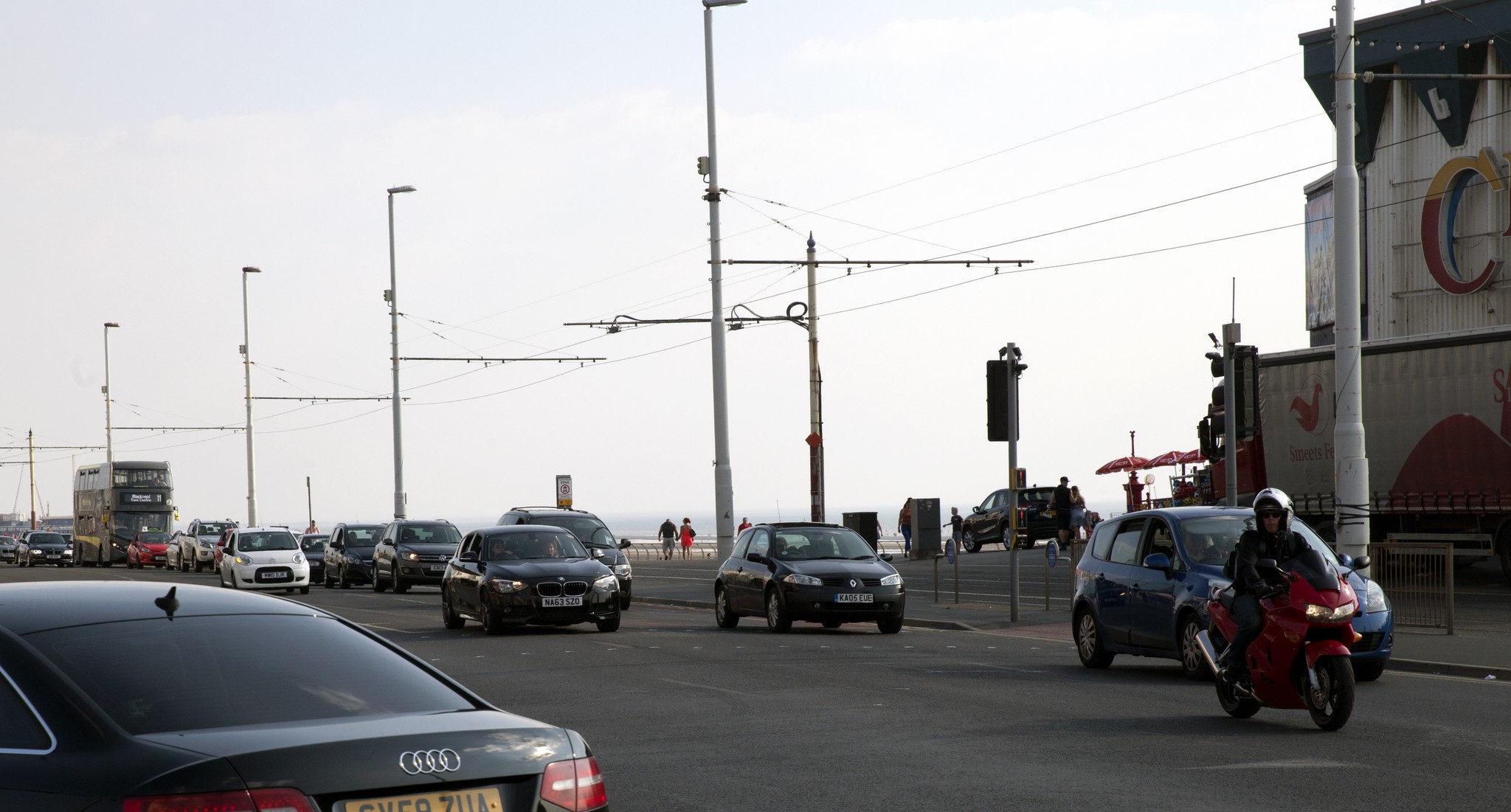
[767,537,787,556]
[1045,476,1079,551]
[737,517,751,538]
[0,473,508,560]
[1070,486,1093,550]
[658,519,678,560]
[543,539,565,558]
[885,529,897,536]
[675,518,696,560]
[898,497,913,558]
[942,506,965,555]
[1223,488,1314,678]
[748,523,752,527]
[707,553,711,557]
[877,519,883,539]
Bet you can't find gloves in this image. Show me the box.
[1255,583,1276,599]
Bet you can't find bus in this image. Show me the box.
[73,461,180,569]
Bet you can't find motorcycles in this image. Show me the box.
[1196,516,1373,733]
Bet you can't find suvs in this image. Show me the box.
[322,522,389,589]
[496,506,633,611]
[962,486,1074,553]
[178,518,240,573]
[371,516,463,593]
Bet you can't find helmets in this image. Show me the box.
[1253,487,1294,529]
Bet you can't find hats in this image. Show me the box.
[1060,477,1070,482]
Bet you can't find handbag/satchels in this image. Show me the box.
[690,525,696,537]
[1084,507,1093,525]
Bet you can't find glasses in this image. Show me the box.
[494,545,504,549]
[1262,511,1280,519]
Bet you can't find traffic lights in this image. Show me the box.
[1199,421,1218,457]
[1011,468,1027,530]
[1202,353,1247,440]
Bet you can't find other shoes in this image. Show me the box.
[904,554,908,557]
[665,556,668,560]
[1059,546,1067,550]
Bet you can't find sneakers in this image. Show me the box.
[1223,665,1238,682]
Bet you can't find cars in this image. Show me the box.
[164,530,187,571]
[0,528,74,568]
[1069,506,1396,685]
[441,524,621,635]
[0,578,608,812]
[296,533,331,584]
[126,526,172,569]
[715,522,906,635]
[219,527,311,595]
[289,529,305,540]
[213,527,242,574]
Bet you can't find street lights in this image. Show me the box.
[386,185,418,519]
[240,265,262,522]
[699,0,751,569]
[102,321,122,460]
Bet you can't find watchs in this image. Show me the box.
[898,527,900,529]
